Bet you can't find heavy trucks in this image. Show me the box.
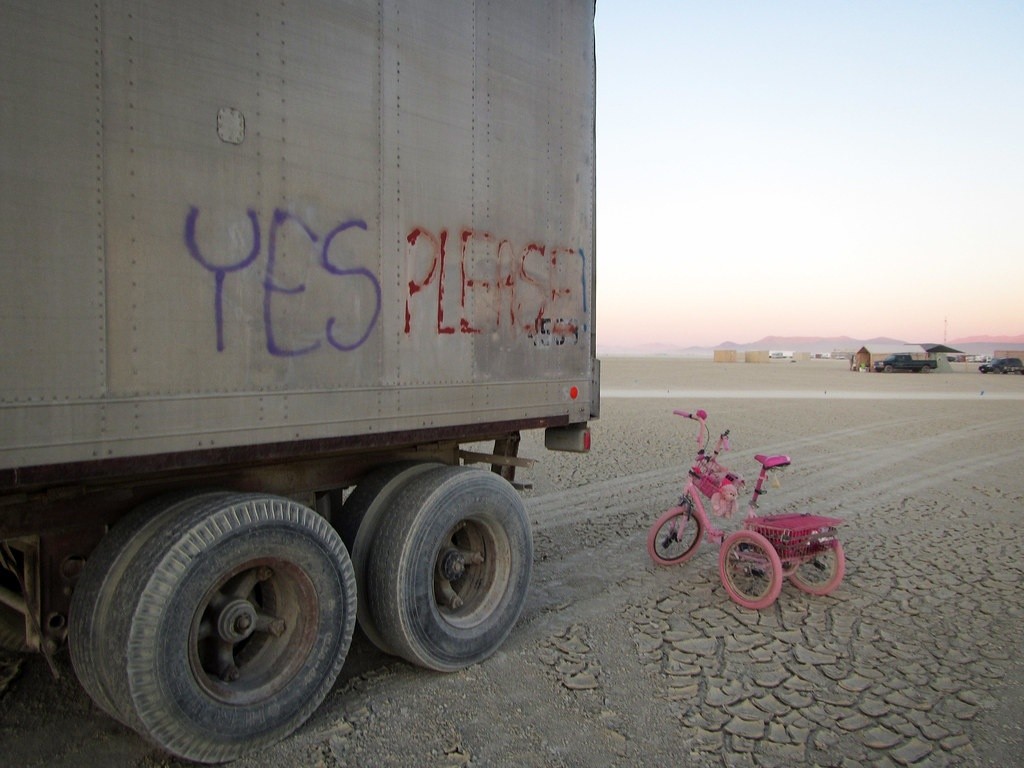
[2,0,604,768]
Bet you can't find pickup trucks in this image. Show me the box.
[873,352,939,375]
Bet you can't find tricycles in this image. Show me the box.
[647,407,851,610]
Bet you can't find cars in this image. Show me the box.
[978,356,1024,375]
